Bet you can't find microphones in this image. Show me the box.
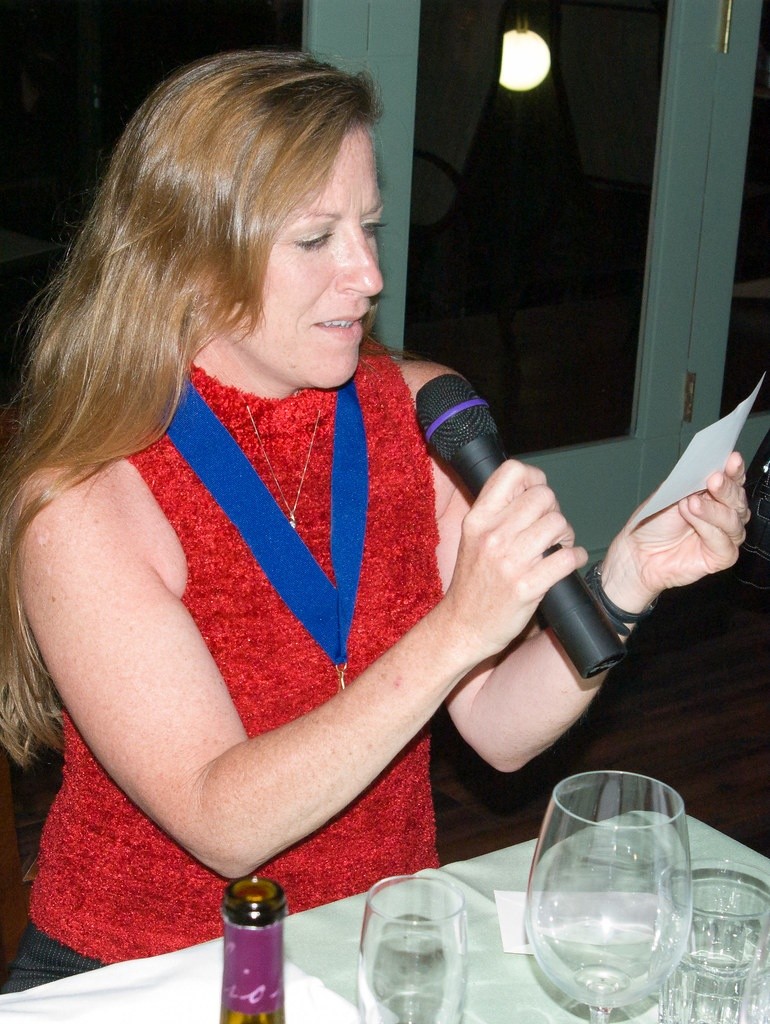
[415,374,629,679]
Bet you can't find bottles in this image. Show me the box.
[220,876,286,1024]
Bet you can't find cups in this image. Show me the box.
[659,860,770,1024]
[357,875,467,1024]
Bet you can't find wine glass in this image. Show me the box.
[525,771,693,1024]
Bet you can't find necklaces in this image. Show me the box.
[206,338,324,529]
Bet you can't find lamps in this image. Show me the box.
[498,0,553,94]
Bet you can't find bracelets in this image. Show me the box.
[583,559,657,637]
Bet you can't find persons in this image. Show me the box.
[1,49,751,998]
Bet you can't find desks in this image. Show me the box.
[0,810,770,1024]
